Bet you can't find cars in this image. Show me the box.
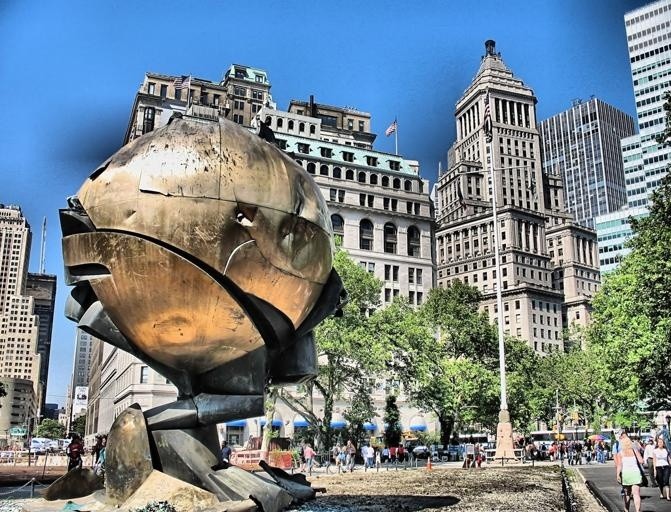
[29,438,72,454]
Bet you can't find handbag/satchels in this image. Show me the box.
[640,471,648,487]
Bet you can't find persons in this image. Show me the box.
[66,435,107,472]
[514,425,610,465]
[612,432,671,512]
[221,441,231,463]
[297,440,415,468]
[463,443,486,468]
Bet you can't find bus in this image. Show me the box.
[530,428,626,459]
[404,434,489,461]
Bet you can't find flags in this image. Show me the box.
[482,86,493,143]
[176,76,190,89]
[384,119,396,137]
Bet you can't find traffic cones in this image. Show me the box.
[426,456,433,472]
